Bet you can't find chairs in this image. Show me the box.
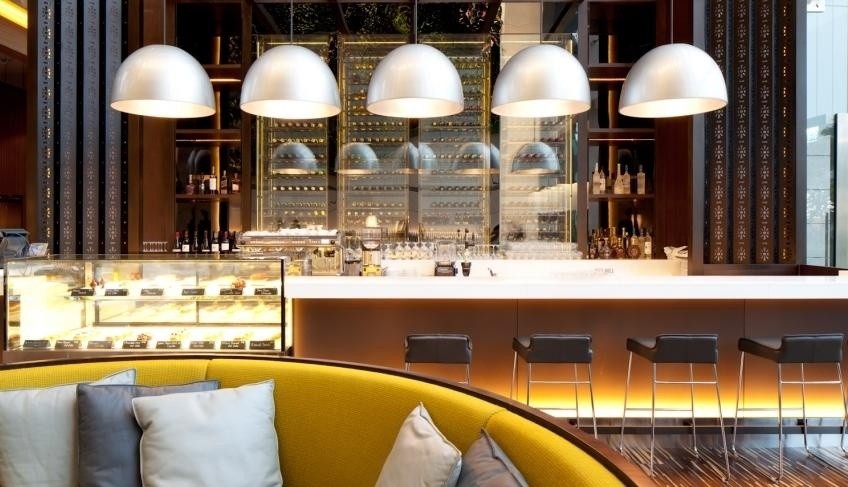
[404,333,472,386]
[512,332,600,439]
[734,330,848,480]
[618,332,732,479]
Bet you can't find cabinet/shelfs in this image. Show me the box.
[576,1,656,255]
[163,0,251,251]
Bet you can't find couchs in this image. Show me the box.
[0,353,662,486]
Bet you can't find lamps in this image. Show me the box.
[268,136,565,180]
[103,0,727,119]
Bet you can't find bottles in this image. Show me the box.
[588,226,652,260]
[186,165,240,193]
[591,161,646,194]
[172,229,239,253]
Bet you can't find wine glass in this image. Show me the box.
[473,242,496,258]
[344,234,362,259]
[384,239,434,260]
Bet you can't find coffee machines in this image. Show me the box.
[432,239,458,275]
[359,226,386,275]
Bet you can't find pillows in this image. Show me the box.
[372,401,463,486]
[131,377,286,486]
[455,426,528,486]
[0,367,137,486]
[75,378,221,486]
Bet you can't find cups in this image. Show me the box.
[501,240,584,258]
